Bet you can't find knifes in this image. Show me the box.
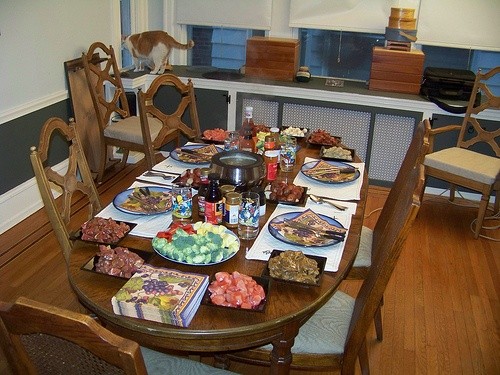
[284,217,344,242]
[128,187,159,211]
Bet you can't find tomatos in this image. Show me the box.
[156,221,197,243]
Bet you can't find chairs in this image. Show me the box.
[137,73,202,171]
[423,64,500,239]
[343,119,432,342]
[82,41,164,185]
[30,117,104,262]
[213,164,425,375]
[67,138,368,375]
[0,296,242,375]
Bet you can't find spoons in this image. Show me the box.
[310,194,349,211]
[143,172,175,180]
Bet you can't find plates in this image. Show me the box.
[250,180,308,206]
[113,186,178,216]
[200,270,270,314]
[68,217,138,247]
[262,250,328,288]
[152,224,241,266]
[268,212,346,248]
[169,125,360,185]
[79,245,155,281]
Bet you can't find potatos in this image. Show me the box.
[194,221,239,252]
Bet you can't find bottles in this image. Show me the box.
[180,167,242,228]
[223,107,281,182]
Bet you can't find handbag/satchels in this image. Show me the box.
[420,66,481,115]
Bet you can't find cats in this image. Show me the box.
[121,30,195,75]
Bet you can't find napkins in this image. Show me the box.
[305,197,358,215]
[128,212,173,238]
[136,170,180,186]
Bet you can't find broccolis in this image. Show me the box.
[256,134,284,149]
[151,229,230,264]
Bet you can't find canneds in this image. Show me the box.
[265,151,278,183]
[221,185,242,228]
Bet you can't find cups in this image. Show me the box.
[280,136,297,173]
[171,182,193,223]
[236,191,259,240]
